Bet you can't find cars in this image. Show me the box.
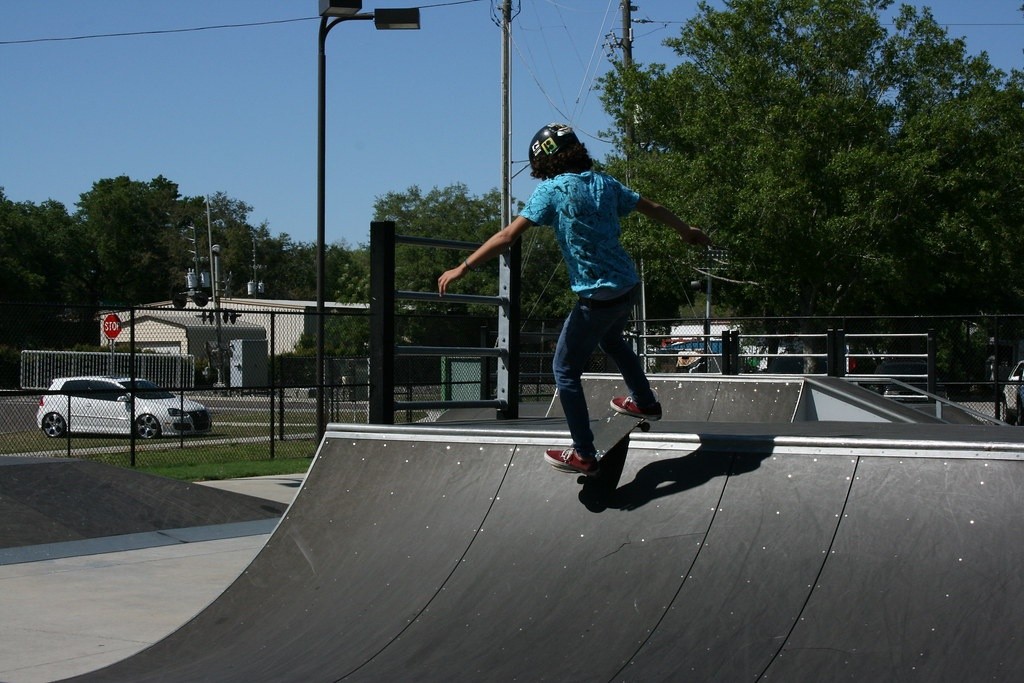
[35,375,213,440]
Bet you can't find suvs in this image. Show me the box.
[869,358,952,403]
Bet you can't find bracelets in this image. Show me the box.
[464,260,471,270]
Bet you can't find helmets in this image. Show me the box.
[529,122,576,170]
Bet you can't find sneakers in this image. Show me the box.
[609,397,663,421]
[543,444,600,476]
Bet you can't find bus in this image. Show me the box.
[656,335,724,359]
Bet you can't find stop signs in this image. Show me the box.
[104,315,123,339]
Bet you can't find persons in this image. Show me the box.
[437,124,715,474]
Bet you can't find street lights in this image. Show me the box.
[623,98,651,373]
[309,1,422,449]
[211,242,230,389]
[686,244,729,372]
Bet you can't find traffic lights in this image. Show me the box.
[690,280,708,292]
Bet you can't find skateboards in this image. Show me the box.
[551,386,660,475]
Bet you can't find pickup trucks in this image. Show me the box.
[1000,359,1024,426]
